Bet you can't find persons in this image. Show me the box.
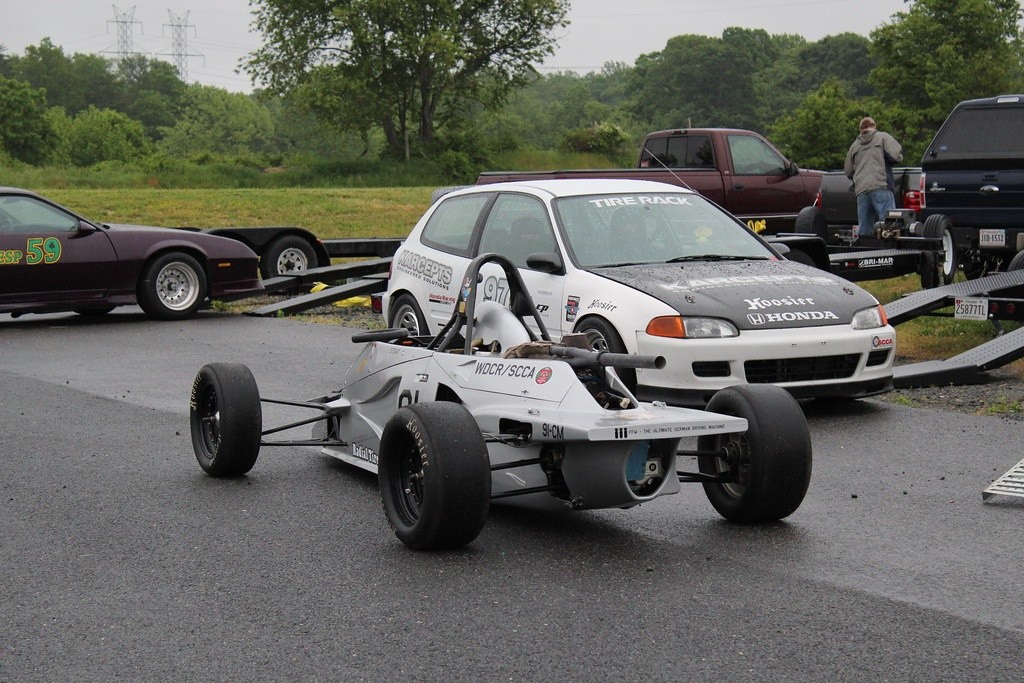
[844,117,904,235]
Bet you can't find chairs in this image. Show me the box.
[609,205,648,254]
[510,217,548,239]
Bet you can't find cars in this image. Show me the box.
[0,185,265,321]
[371,177,900,417]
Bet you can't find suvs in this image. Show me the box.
[915,91,1024,265]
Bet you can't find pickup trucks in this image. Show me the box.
[476,126,838,239]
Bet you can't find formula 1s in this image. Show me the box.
[189,253,814,551]
